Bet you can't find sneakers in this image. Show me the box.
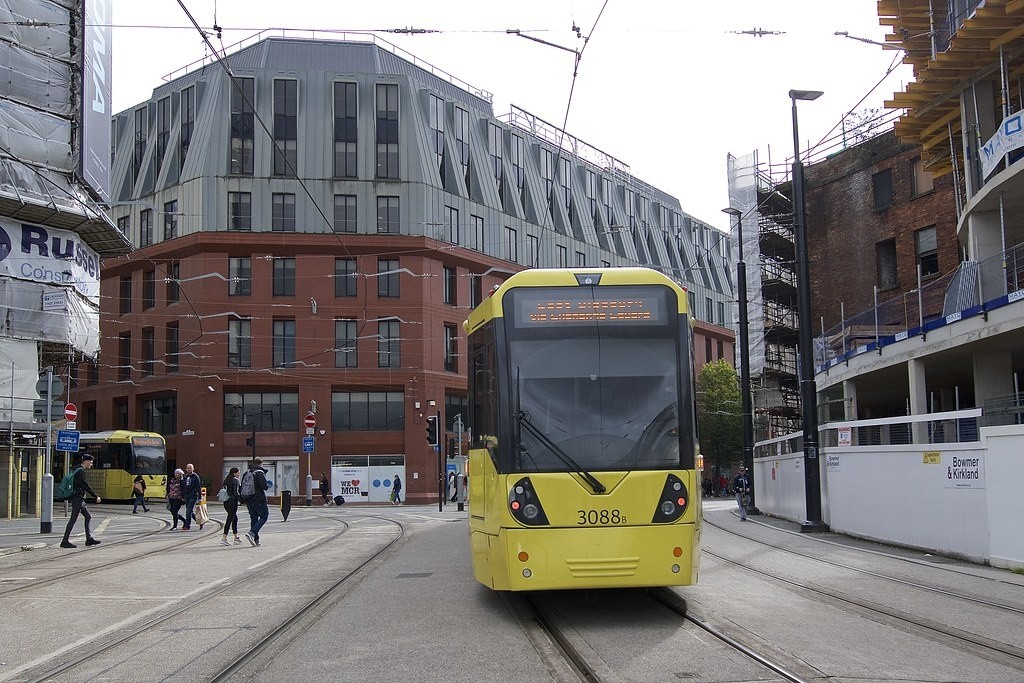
[233,537,243,544]
[252,542,261,546]
[219,539,231,546]
[245,533,256,546]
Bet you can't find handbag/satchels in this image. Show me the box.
[390,490,395,501]
[195,504,209,524]
[134,482,143,495]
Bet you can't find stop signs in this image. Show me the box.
[65,404,78,422]
[304,414,316,428]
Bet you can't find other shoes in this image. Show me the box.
[132,512,138,514]
[181,526,191,530]
[60,540,76,548]
[398,502,402,506]
[170,527,177,531]
[391,501,394,505]
[85,538,100,546]
[199,523,206,530]
[145,509,150,513]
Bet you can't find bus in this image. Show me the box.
[49,430,167,503]
[463,268,702,592]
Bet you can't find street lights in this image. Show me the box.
[39,366,54,533]
[453,414,465,512]
[721,206,760,515]
[789,87,829,532]
[306,411,314,507]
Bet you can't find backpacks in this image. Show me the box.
[241,470,265,499]
[57,467,85,500]
[216,485,230,502]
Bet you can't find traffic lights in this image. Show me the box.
[449,438,458,458]
[426,416,438,446]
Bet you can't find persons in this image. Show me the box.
[241,457,269,546]
[391,475,402,505]
[448,472,460,502]
[702,473,736,498]
[166,468,186,530]
[180,463,205,529]
[220,467,244,545]
[319,472,332,506]
[59,453,102,548]
[131,471,150,514]
[732,466,752,519]
